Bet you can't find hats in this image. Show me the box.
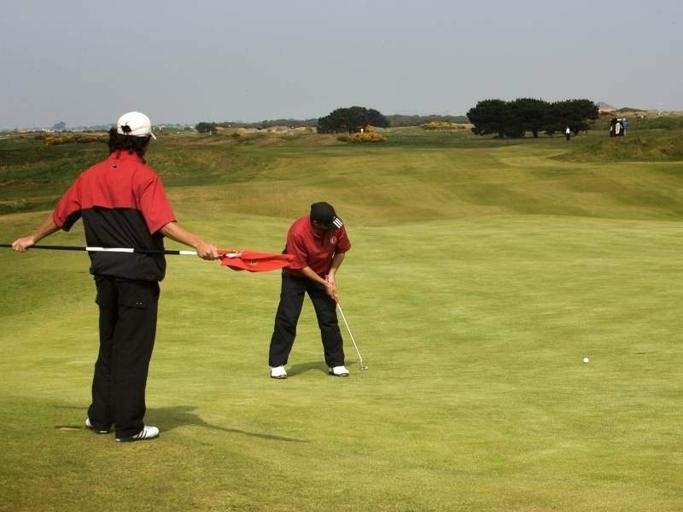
[310,202,343,230]
[118,111,156,140]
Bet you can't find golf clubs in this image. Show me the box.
[325,274,369,373]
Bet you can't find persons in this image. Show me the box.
[265,200,350,379]
[614,121,623,138]
[8,110,224,446]
[565,125,571,141]
[622,117,628,137]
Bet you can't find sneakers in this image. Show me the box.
[329,365,349,376]
[270,365,287,378]
[85,418,108,434]
[115,425,159,442]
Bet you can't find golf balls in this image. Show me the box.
[583,357,588,363]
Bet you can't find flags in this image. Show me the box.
[211,246,297,274]
